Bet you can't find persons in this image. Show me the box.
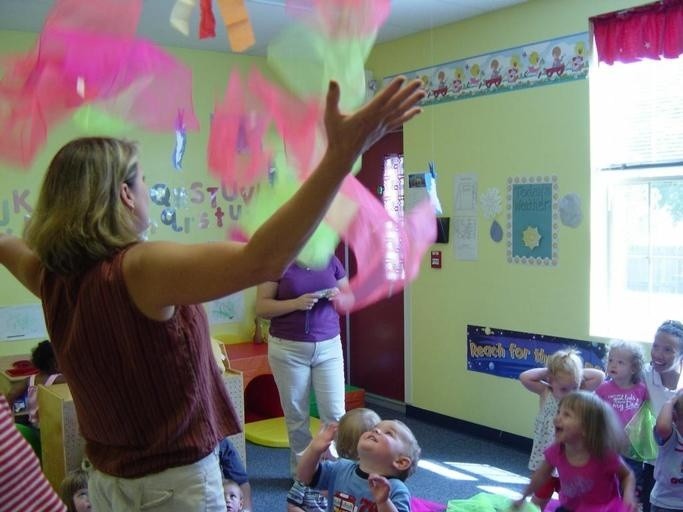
[593,344,651,512]
[7,340,67,430]
[651,389,682,511]
[220,482,244,511]
[0,396,66,512]
[640,320,681,511]
[257,240,356,475]
[288,407,380,511]
[219,436,252,512]
[516,351,607,496]
[514,391,636,512]
[53,470,88,506]
[294,419,421,512]
[2,74,424,512]
